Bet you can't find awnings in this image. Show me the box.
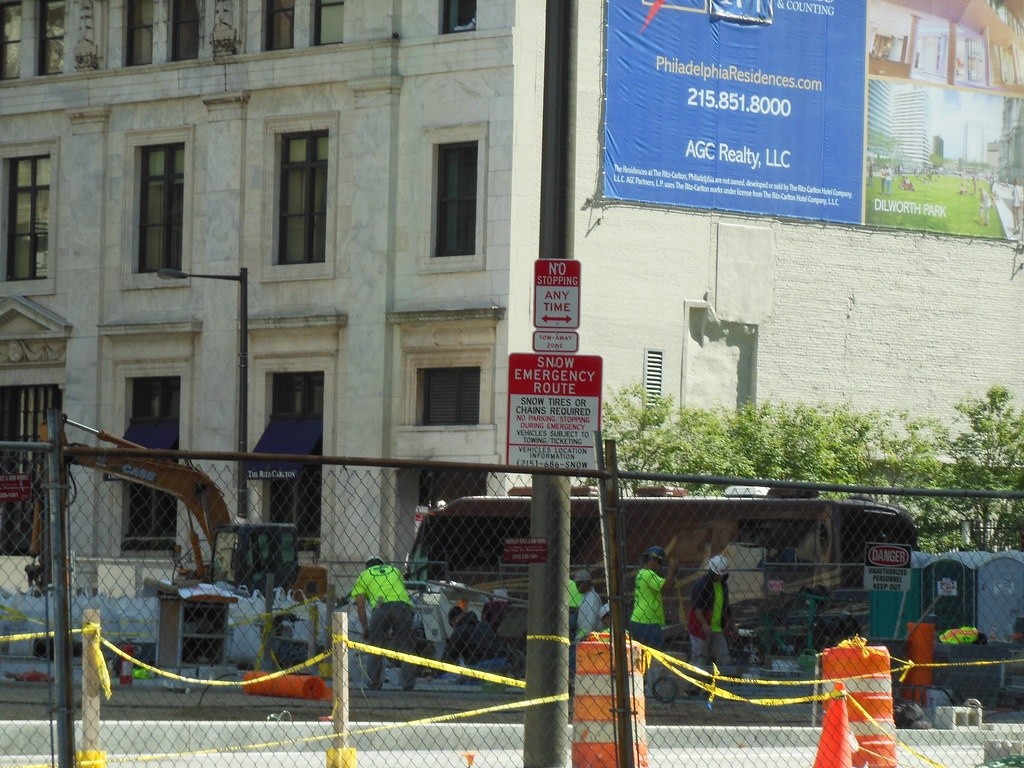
[103,416,179,481]
[246,413,323,480]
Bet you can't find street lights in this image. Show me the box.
[157,266,251,521]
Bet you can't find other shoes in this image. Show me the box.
[367,680,380,690]
[404,685,413,691]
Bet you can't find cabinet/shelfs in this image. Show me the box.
[156,595,230,667]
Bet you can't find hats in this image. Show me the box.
[448,606,462,627]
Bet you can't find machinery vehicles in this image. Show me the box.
[24,411,328,601]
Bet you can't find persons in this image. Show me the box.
[426,605,512,685]
[350,557,418,692]
[630,547,673,693]
[680,554,732,699]
[569,567,632,696]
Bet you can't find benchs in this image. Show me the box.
[934,705,983,730]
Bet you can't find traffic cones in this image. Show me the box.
[812,681,855,768]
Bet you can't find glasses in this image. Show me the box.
[652,557,663,564]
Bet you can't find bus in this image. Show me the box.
[404,486,918,658]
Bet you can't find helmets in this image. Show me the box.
[600,602,609,620]
[709,555,730,575]
[367,555,386,564]
[646,546,666,560]
[574,570,591,582]
